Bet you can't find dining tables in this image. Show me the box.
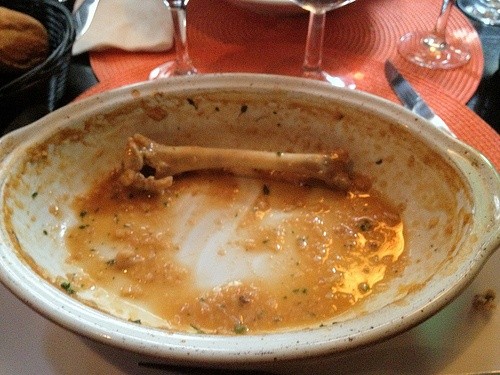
[0,1,500,174]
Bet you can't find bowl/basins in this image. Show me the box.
[0,71,500,367]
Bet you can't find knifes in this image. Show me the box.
[384,58,457,138]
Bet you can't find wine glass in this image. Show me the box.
[281,0,356,90]
[398,0,500,68]
[148,0,220,81]
